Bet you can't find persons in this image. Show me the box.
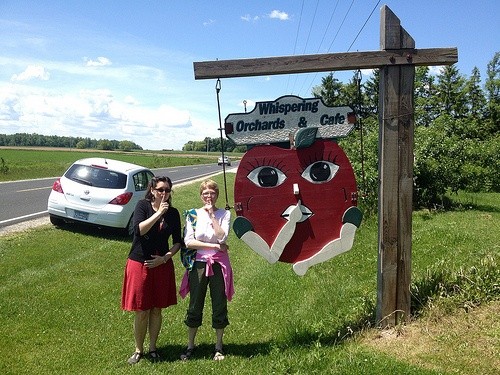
[181,178,231,360]
[121,175,183,364]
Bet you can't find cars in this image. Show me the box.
[217,156,232,166]
[46,157,155,240]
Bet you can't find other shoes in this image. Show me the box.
[213,350,225,362]
[128,352,144,364]
[180,347,195,361]
[148,348,161,362]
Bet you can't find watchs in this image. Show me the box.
[209,213,216,218]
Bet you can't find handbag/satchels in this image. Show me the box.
[181,209,197,272]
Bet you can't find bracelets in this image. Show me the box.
[162,255,167,263]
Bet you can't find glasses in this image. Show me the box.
[155,188,171,193]
